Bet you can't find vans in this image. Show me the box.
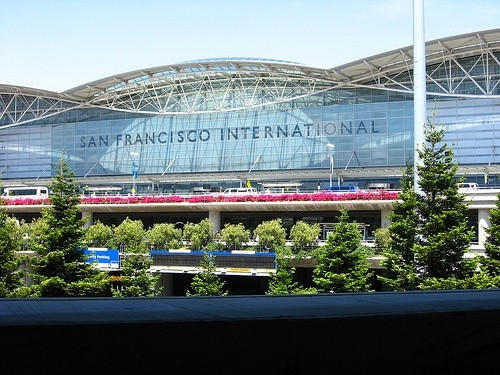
[220,187,257,196]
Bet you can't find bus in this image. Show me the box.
[3,186,48,200]
